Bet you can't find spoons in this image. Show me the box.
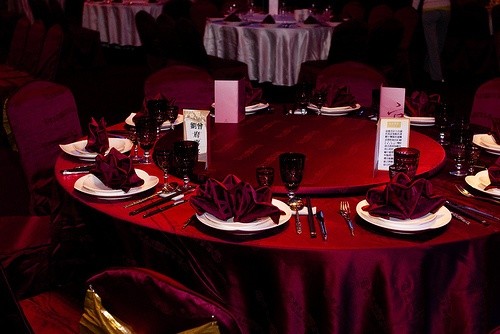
[288,197,305,234]
[122,181,179,208]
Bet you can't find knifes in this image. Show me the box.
[142,198,190,218]
[306,197,317,237]
[317,210,328,241]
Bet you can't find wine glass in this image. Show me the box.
[278,152,305,211]
[223,2,334,28]
[433,102,481,177]
[127,100,201,194]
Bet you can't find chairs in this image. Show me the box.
[78,267,241,334]
[0,18,63,88]
[468,77,500,136]
[368,5,419,63]
[0,262,85,334]
[3,80,82,214]
[142,66,216,109]
[310,62,388,108]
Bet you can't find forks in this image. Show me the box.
[339,200,354,232]
[455,183,500,205]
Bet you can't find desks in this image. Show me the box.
[203,17,341,86]
[81,0,163,46]
[52,103,500,334]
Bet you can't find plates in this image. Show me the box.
[308,103,361,116]
[125,112,185,128]
[355,198,452,231]
[195,198,294,233]
[73,168,159,196]
[59,137,133,161]
[471,133,500,153]
[245,103,269,113]
[410,117,435,127]
[465,170,500,197]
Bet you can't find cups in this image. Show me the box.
[389,147,421,181]
[256,167,274,187]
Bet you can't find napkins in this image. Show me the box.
[312,82,361,109]
[190,174,286,224]
[484,157,500,191]
[361,173,448,220]
[85,116,109,155]
[89,148,145,192]
[404,92,441,116]
